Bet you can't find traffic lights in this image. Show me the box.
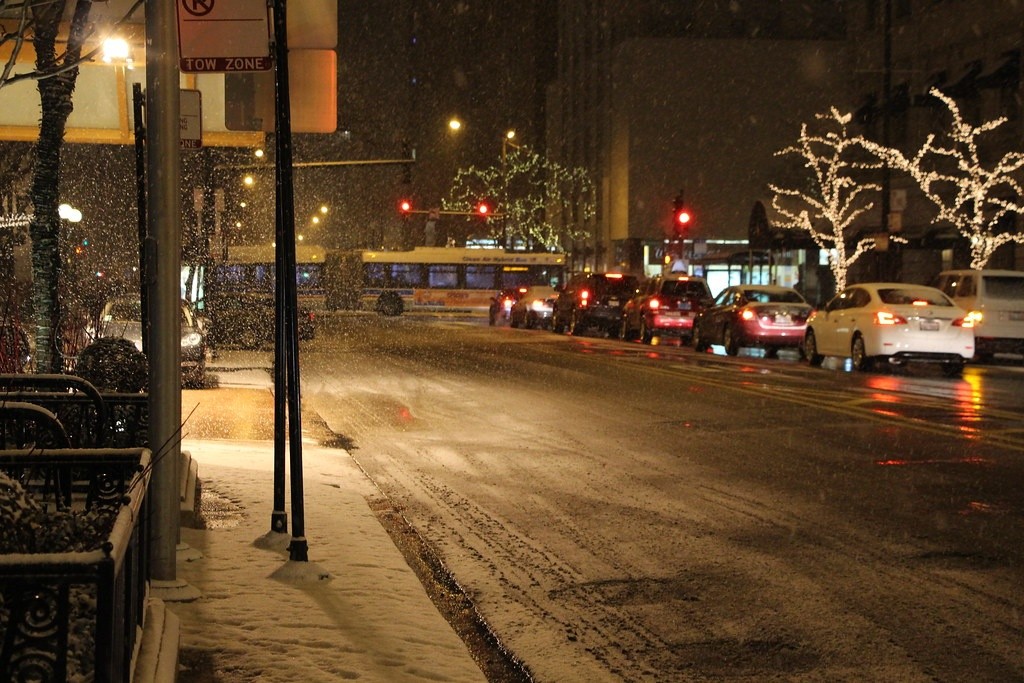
[678,212,689,238]
[479,203,487,222]
[402,200,410,221]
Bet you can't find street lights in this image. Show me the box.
[502,129,516,245]
[58,204,83,316]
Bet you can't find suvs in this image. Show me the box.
[551,271,640,336]
[618,275,714,343]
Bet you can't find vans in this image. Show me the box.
[925,270,1024,361]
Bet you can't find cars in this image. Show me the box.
[91,296,212,386]
[802,282,974,377]
[195,292,315,350]
[510,287,562,330]
[489,285,530,326]
[691,285,816,356]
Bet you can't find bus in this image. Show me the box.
[222,243,565,318]
